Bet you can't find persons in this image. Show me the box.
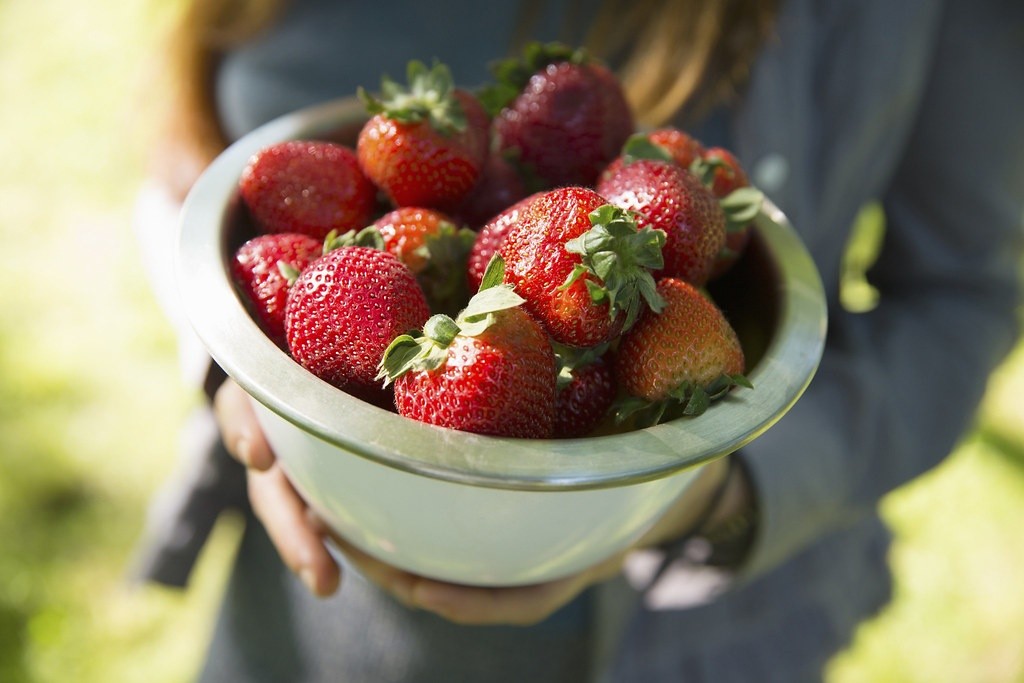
[138,1,1024,683]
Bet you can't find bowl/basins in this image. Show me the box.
[171,94,828,591]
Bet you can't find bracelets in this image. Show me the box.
[673,495,757,557]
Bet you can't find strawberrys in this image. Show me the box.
[235,39,760,438]
